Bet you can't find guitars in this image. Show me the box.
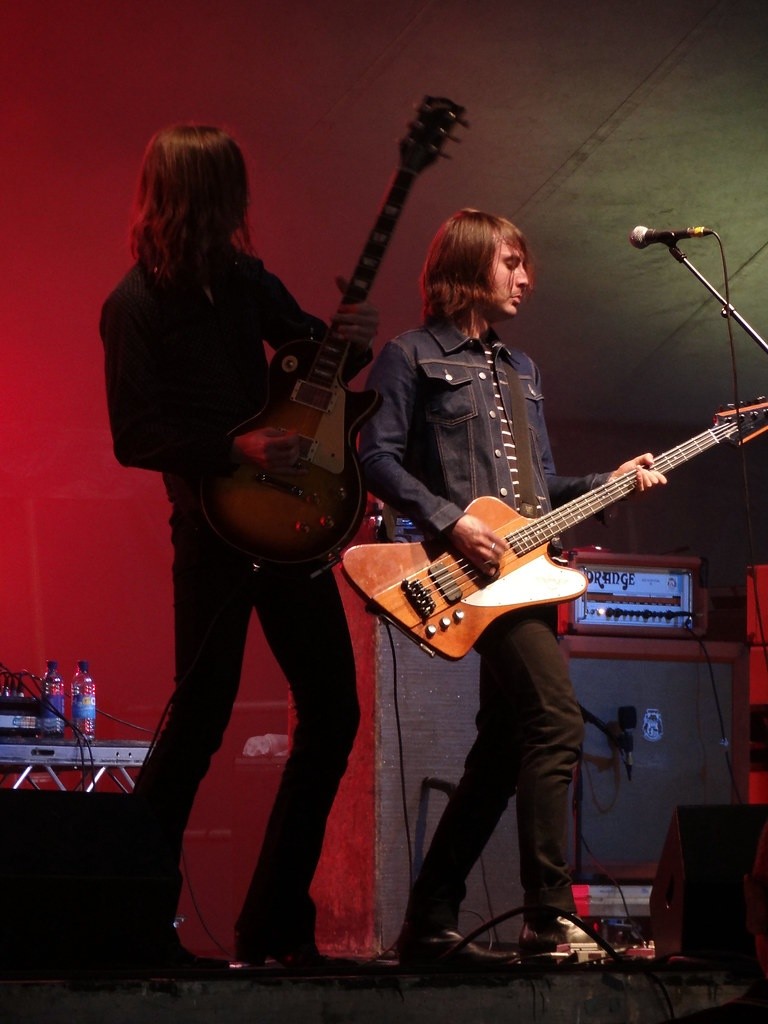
[340,389,768,661]
[197,88,467,571]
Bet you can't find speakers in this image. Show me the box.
[556,635,750,887]
[651,802,768,957]
[368,616,526,949]
[0,787,131,982]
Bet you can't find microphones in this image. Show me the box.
[618,706,637,772]
[630,225,713,249]
[423,776,458,795]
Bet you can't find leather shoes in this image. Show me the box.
[396,928,527,975]
[234,937,366,975]
[68,939,230,975]
[518,914,606,958]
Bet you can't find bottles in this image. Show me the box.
[71,660,96,740]
[41,660,64,738]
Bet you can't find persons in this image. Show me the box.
[101,124,386,989]
[354,204,670,972]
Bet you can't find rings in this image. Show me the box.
[490,542,496,551]
